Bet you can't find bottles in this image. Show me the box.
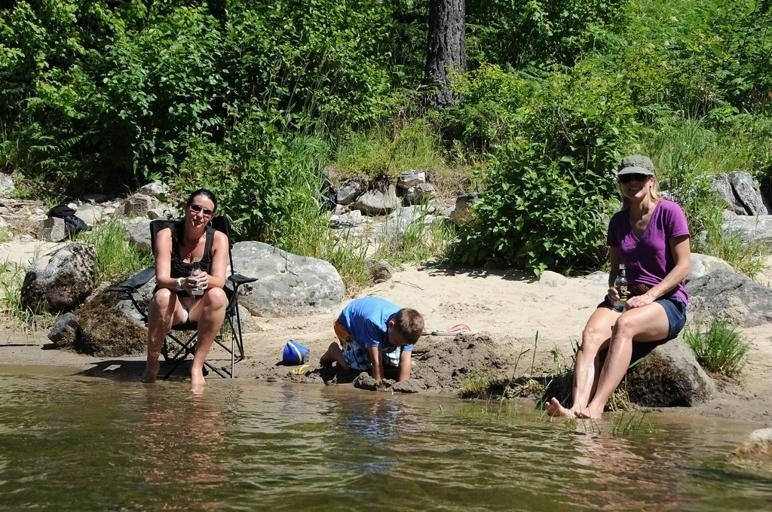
[190,262,204,295]
[614,263,628,313]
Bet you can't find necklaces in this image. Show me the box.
[183,245,196,258]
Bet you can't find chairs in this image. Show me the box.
[105,215,258,386]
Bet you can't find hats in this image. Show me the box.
[617,155,656,177]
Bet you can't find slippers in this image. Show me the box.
[433,324,479,335]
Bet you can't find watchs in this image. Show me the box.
[175,277,185,291]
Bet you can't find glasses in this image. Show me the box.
[620,174,648,184]
[190,203,212,215]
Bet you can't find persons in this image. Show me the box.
[319,296,425,383]
[138,188,229,385]
[544,155,692,420]
